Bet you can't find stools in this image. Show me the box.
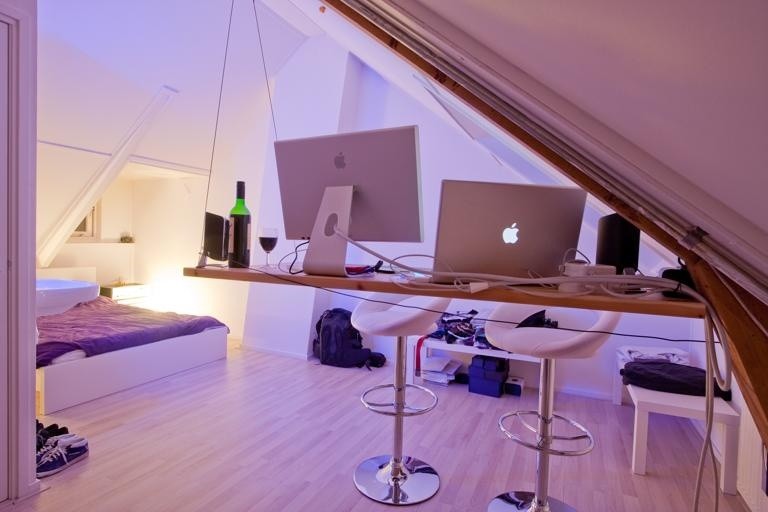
[485,303,620,512]
[352,291,451,504]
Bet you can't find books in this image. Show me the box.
[422,356,464,387]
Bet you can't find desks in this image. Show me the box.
[182,261,768,447]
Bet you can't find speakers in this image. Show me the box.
[197,212,229,268]
[596,213,640,275]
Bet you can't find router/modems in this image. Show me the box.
[565,261,617,278]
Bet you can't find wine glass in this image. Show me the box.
[258,226,280,270]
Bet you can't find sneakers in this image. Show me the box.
[35,419,89,478]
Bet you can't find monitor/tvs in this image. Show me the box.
[274,125,424,277]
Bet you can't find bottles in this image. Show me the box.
[227,181,251,269]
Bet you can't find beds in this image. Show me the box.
[35,298,227,415]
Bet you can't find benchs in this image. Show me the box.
[612,347,738,495]
[404,331,541,387]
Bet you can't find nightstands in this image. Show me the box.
[99,282,144,307]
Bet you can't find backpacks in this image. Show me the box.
[313,307,371,368]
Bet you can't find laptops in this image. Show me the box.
[433,180,587,284]
